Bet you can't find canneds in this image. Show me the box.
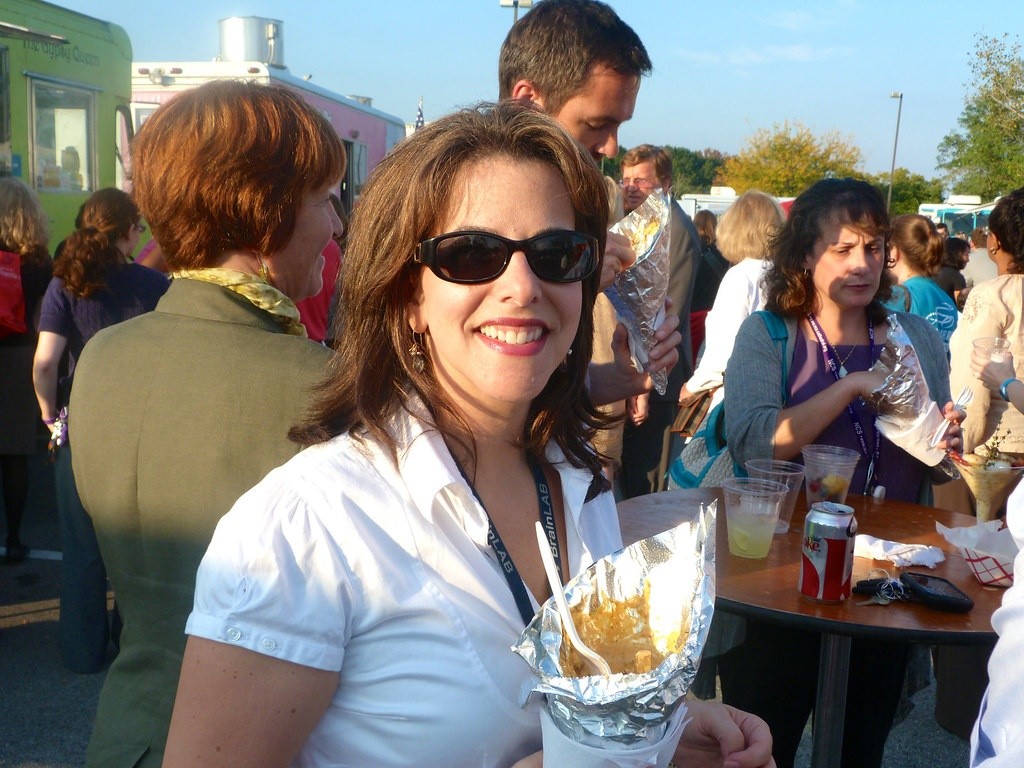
[798,501,857,605]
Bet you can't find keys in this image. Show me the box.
[856,595,891,606]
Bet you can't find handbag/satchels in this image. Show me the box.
[670,390,713,436]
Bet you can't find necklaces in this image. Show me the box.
[822,326,864,379]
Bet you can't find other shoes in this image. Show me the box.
[2,542,28,560]
[97,640,117,657]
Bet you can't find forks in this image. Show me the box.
[931,387,973,447]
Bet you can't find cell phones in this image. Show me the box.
[900,571,974,613]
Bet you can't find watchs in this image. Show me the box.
[999,378,1022,402]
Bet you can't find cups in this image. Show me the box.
[801,445,861,512]
[745,459,808,533]
[973,337,1011,384]
[719,478,790,558]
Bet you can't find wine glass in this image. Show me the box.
[950,455,1024,526]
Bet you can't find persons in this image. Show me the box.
[0,175,53,564]
[32,187,172,676]
[54,201,171,278]
[296,193,360,350]
[163,98,776,768]
[500,0,682,406]
[880,187,1024,768]
[590,144,788,504]
[689,177,966,768]
[67,79,389,768]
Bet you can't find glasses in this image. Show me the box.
[411,229,600,284]
[619,176,662,189]
[135,225,145,233]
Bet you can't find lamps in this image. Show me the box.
[150,69,162,84]
[353,131,359,138]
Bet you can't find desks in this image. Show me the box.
[615,486,1021,768]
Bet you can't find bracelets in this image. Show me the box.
[42,415,58,424]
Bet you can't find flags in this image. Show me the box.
[415,107,424,130]
